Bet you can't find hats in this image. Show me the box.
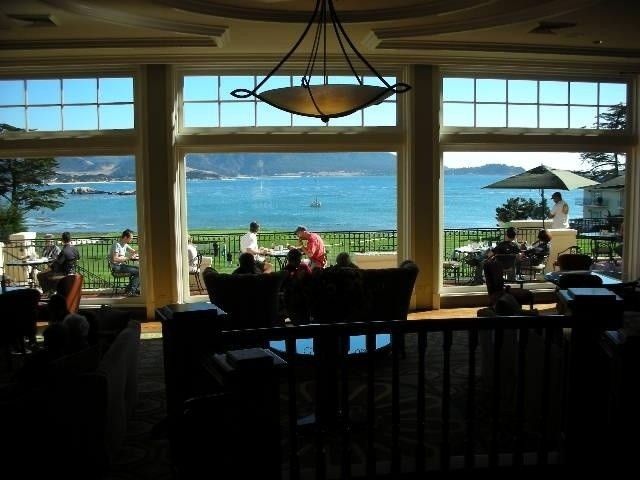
[550,192,561,199]
[250,220,260,231]
[293,225,306,235]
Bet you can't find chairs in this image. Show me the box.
[0,289,141,460]
[108,256,138,296]
[0,266,14,286]
[45,258,77,299]
[189,255,204,295]
[320,249,329,269]
[37,274,84,326]
[444,225,631,301]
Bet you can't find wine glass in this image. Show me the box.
[467,240,488,252]
[261,242,284,254]
[612,226,616,235]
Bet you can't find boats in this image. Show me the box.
[69,186,109,196]
[309,195,320,208]
[113,189,138,196]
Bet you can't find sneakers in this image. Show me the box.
[471,279,483,285]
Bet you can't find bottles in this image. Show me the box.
[520,240,532,251]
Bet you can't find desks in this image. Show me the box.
[6,259,54,289]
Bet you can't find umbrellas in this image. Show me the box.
[480,163,601,228]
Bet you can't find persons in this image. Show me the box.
[0,312,90,402]
[548,192,570,229]
[42,234,61,265]
[110,229,140,297]
[187,235,198,273]
[37,232,80,298]
[232,222,359,280]
[516,229,552,275]
[471,227,522,285]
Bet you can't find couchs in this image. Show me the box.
[203,260,419,324]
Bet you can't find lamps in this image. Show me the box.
[230,0,413,123]
